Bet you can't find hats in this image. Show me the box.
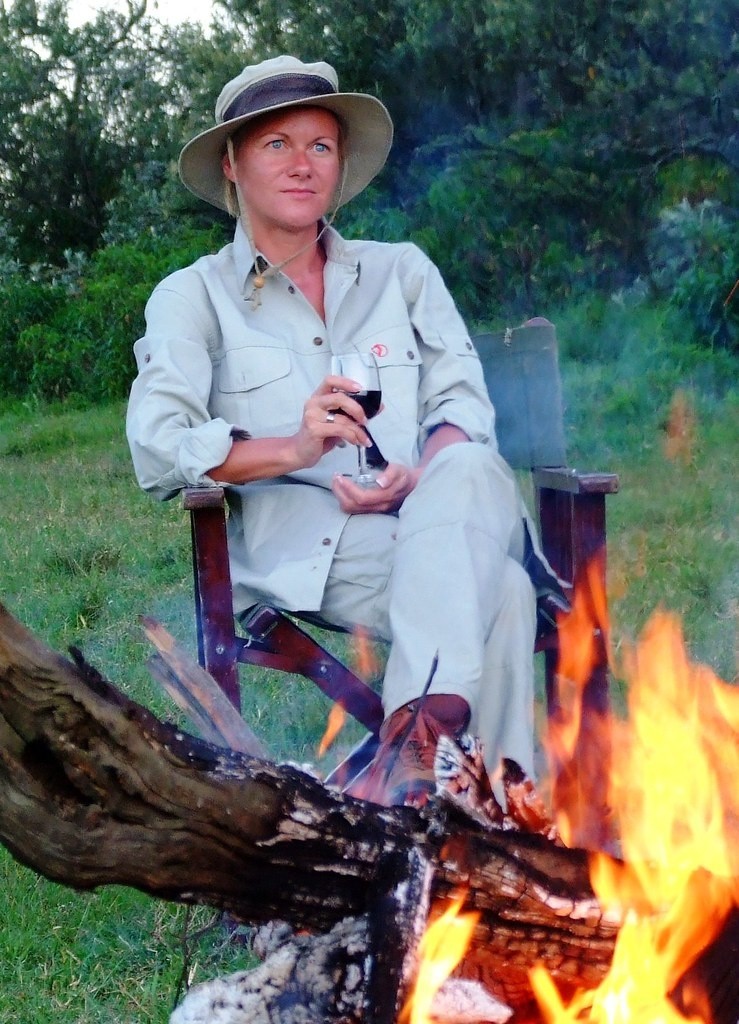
[177,55,393,217]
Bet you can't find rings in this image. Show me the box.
[327,413,337,424]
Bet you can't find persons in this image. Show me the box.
[125,54,535,811]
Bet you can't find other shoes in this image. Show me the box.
[384,731,440,809]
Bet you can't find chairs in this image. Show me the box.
[181,316,618,739]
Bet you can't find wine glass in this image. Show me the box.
[328,353,385,484]
